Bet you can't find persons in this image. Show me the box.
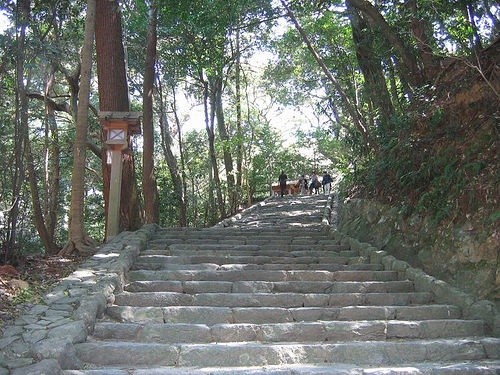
[299,170,334,197]
[278,170,288,197]
